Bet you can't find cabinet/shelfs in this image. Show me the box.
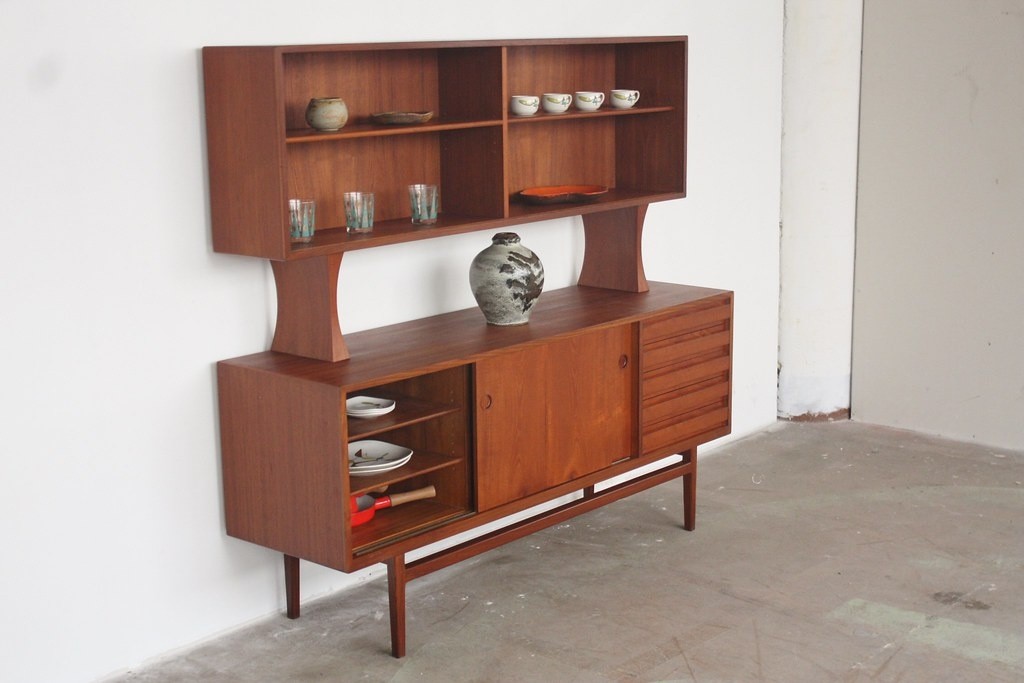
[200,35,735,660]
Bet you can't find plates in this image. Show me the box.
[520,185,609,203]
[369,110,433,123]
[346,395,395,418]
[348,440,413,476]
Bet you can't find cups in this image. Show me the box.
[610,90,639,109]
[573,92,604,111]
[408,184,439,226]
[343,192,375,234]
[289,199,315,242]
[541,94,572,113]
[511,95,540,115]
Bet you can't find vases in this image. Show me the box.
[468,232,544,326]
